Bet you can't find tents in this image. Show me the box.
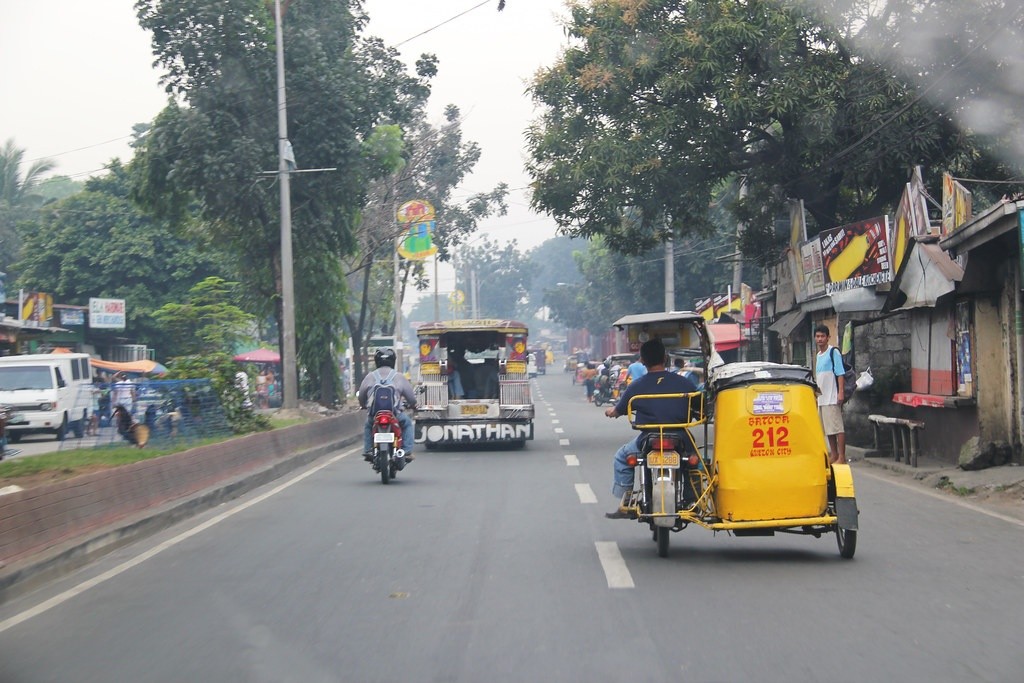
[50,347,171,381]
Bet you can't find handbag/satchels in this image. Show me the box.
[830,346,857,413]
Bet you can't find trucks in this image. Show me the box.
[414,320,536,450]
[0,353,95,441]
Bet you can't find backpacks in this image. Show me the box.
[366,369,399,417]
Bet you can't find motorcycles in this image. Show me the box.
[603,310,860,559]
[355,386,426,485]
[593,384,614,406]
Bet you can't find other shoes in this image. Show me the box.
[683,499,700,510]
[362,453,373,461]
[405,453,416,460]
[605,502,637,520]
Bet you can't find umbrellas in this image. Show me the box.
[234,346,280,373]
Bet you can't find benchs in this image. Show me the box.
[868,415,925,469]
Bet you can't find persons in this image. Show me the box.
[358,348,417,463]
[448,354,464,399]
[86,370,135,437]
[813,325,845,464]
[605,339,705,520]
[570,348,696,404]
[233,368,277,411]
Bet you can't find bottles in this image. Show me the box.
[699,306,713,320]
[716,294,756,318]
[894,205,906,272]
[827,221,882,283]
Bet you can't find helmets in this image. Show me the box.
[374,347,397,369]
